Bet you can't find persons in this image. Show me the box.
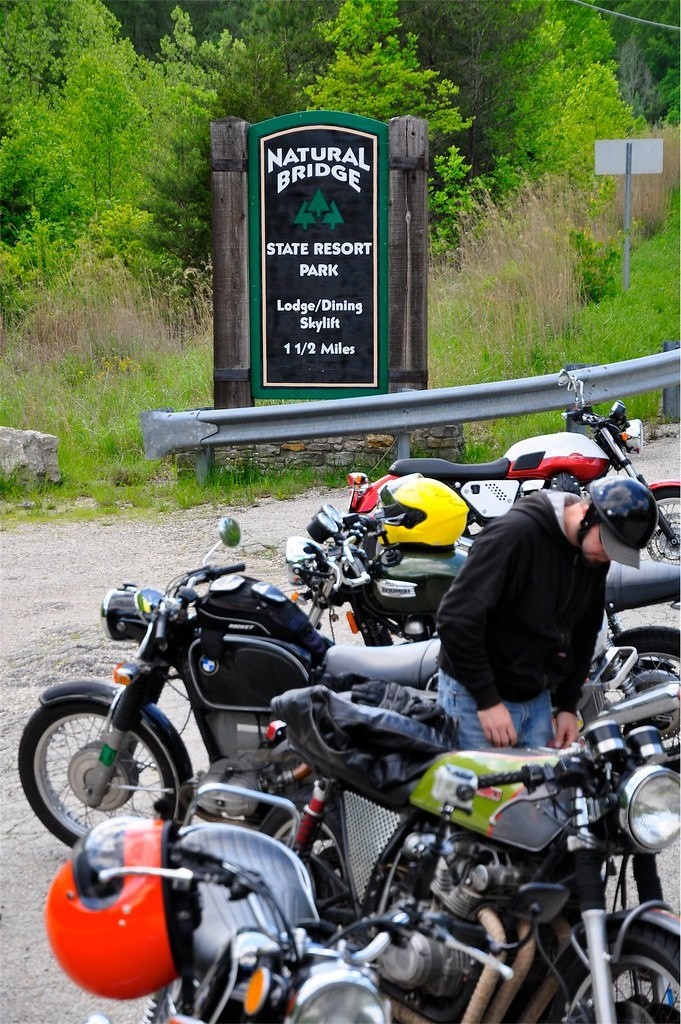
[435,474,657,748]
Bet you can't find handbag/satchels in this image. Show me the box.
[270,680,459,808]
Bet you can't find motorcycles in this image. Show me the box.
[14,360,681,1023]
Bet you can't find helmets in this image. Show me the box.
[588,476,658,549]
[45,814,195,1001]
[373,472,470,551]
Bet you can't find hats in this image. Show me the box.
[599,520,640,570]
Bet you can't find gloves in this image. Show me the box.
[351,680,443,722]
